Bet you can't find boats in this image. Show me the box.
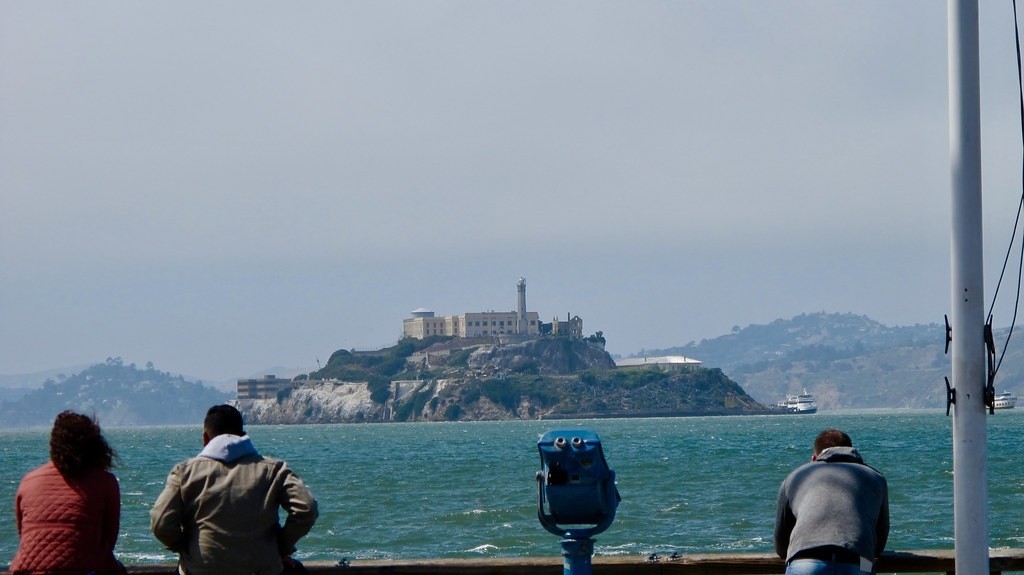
[778,387,817,415]
[986,389,1018,409]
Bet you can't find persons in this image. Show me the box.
[8,410,125,575]
[773,428,891,575]
[151,404,319,575]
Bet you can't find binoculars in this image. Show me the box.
[534,430,623,540]
